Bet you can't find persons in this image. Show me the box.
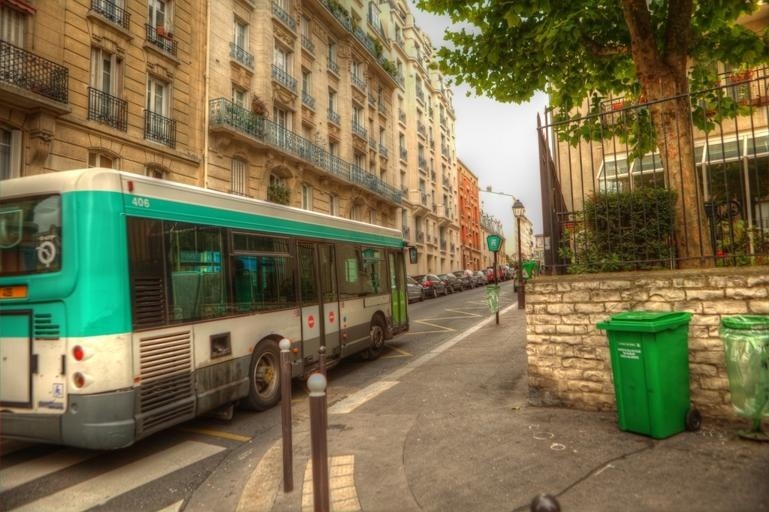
[236,260,254,303]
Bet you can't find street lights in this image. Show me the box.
[511,197,527,310]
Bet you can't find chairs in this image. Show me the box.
[170,270,202,321]
[199,272,223,316]
[236,269,254,311]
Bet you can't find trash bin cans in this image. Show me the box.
[596,310,702,441]
[486,286,501,313]
[720,315,769,441]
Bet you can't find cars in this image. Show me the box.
[404,276,424,302]
[453,270,477,288]
[473,264,512,286]
[438,274,463,292]
[413,273,447,297]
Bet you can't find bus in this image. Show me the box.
[0,166,418,452]
